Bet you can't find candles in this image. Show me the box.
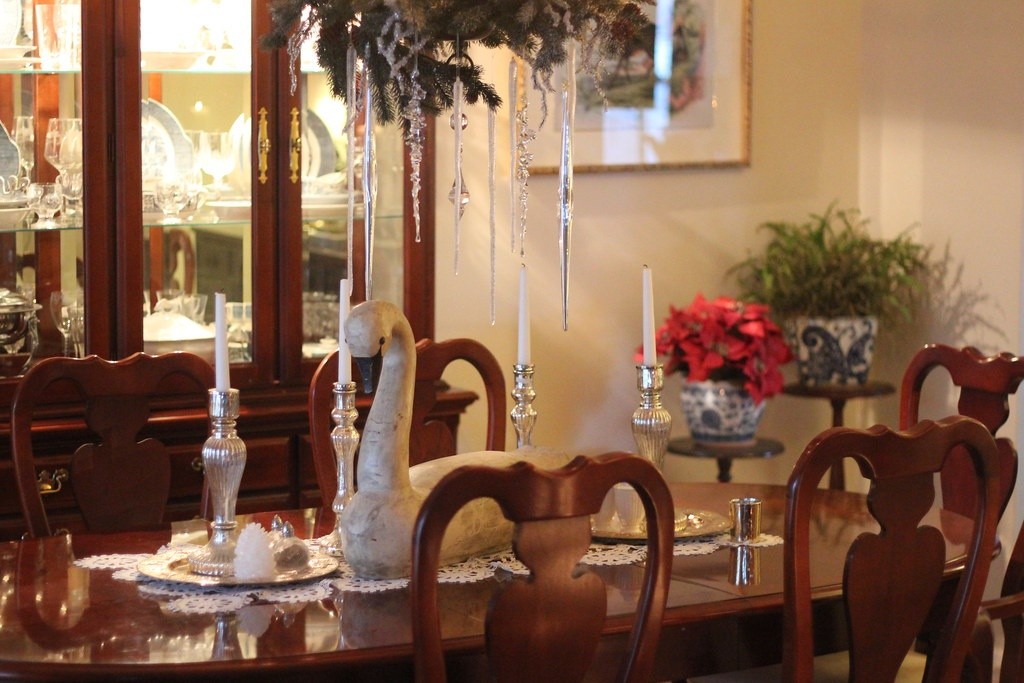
[517,267,532,363]
[213,289,232,394]
[338,278,355,380]
[639,265,658,369]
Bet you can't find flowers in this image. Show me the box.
[634,294,783,398]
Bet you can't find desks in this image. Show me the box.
[0,483,1024,683]
[669,433,786,486]
[775,379,897,491]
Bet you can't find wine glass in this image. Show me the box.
[50,288,340,359]
[10,115,235,227]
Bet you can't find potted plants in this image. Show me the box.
[727,199,934,385]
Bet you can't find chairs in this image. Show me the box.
[785,410,1002,683]
[307,338,512,503]
[409,454,677,683]
[10,352,214,536]
[901,342,1024,683]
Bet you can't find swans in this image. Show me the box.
[345,300,615,581]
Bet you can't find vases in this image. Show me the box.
[676,380,768,443]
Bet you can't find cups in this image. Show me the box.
[728,498,761,543]
[726,547,761,586]
[593,483,645,534]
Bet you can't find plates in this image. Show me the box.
[144,99,192,201]
[589,507,733,543]
[203,195,364,221]
[300,107,332,188]
[137,547,340,585]
[0,44,43,70]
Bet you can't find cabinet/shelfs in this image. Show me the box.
[0,1,479,545]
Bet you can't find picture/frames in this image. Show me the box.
[518,1,753,172]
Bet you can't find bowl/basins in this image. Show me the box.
[142,50,205,70]
